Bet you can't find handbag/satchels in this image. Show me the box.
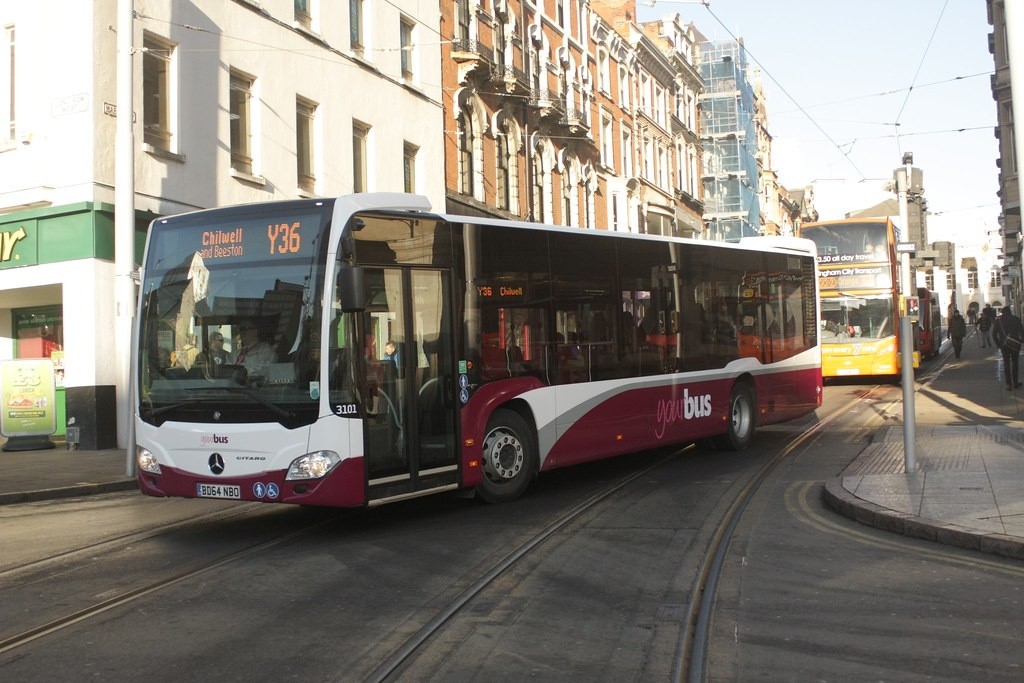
[1003,333,1023,354]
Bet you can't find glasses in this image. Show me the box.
[214,338,224,343]
[238,325,257,332]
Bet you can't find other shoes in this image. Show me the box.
[1006,387,1012,391]
[1014,383,1020,388]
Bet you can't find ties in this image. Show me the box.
[235,347,250,366]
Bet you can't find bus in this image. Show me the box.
[792,214,923,381]
[914,287,943,363]
[130,184,824,511]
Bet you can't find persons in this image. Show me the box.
[842,324,855,336]
[967,308,976,324]
[171,334,200,370]
[195,331,230,364]
[865,243,873,252]
[554,330,584,365]
[226,319,277,376]
[992,306,1024,391]
[384,340,398,365]
[947,310,967,359]
[975,313,991,347]
